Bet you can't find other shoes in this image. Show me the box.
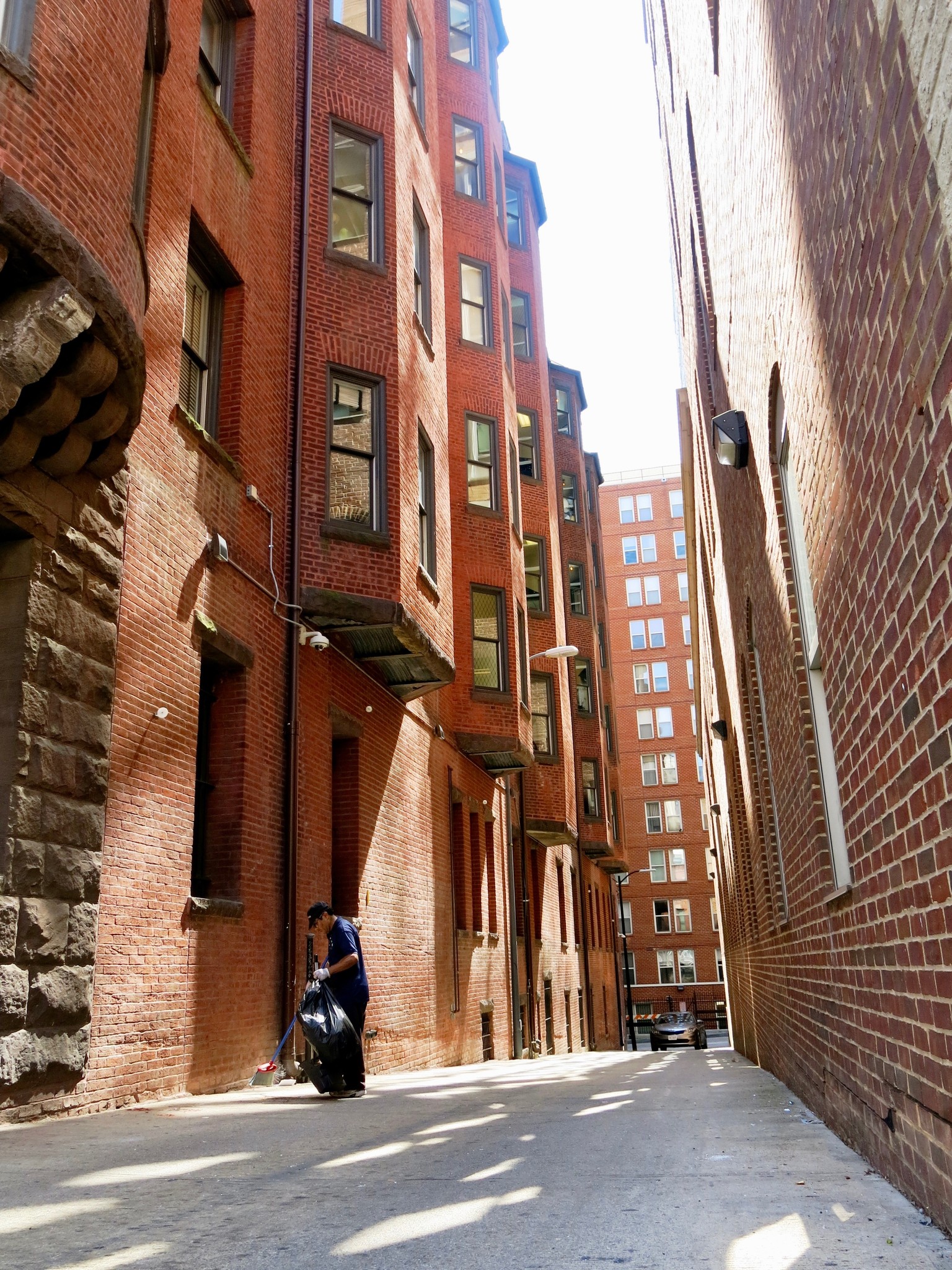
[329,1082,367,1098]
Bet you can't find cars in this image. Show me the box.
[648,1011,708,1051]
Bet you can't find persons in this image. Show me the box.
[307,900,370,1096]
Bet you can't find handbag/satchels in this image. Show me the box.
[295,976,362,1070]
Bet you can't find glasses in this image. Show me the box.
[312,912,323,930]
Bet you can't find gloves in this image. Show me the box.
[313,967,331,981]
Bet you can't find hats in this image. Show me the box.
[307,901,330,930]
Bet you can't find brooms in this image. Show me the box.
[247,1016,299,1087]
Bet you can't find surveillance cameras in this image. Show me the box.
[309,635,330,651]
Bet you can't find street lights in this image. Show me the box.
[617,868,657,1051]
